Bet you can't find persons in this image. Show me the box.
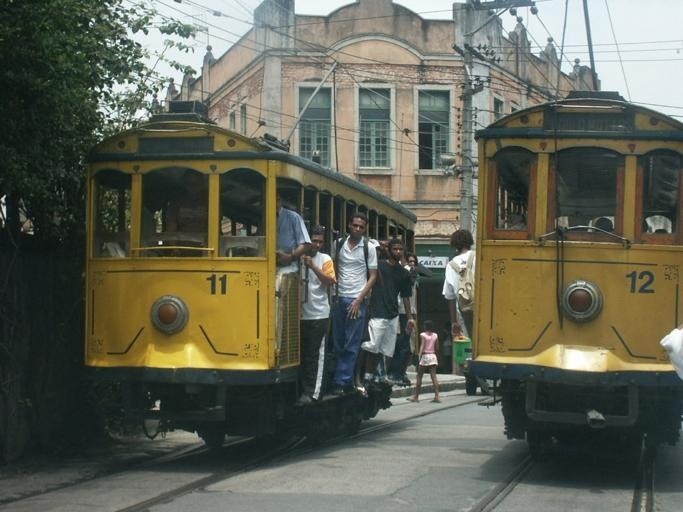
[441,230,476,340]
[276,192,441,403]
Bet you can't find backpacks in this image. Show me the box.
[449,250,476,314]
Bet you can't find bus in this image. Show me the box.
[82,99,419,446]
[462,87,683,462]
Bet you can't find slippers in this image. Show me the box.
[405,396,420,403]
[431,399,442,403]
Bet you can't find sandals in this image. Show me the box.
[357,385,369,398]
